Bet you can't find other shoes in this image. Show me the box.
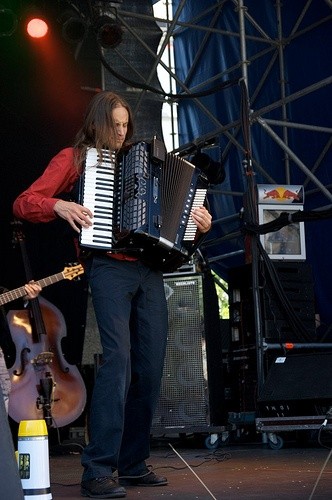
[80,476,127,499]
[118,469,168,487]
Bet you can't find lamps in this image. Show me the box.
[0,8,18,37]
[58,10,86,43]
[95,15,123,49]
[192,149,226,185]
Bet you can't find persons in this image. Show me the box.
[13,91,212,500]
[0,281,44,370]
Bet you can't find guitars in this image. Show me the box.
[0,261,85,307]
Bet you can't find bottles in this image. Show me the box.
[16,419,54,499]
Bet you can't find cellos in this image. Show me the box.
[5,218,87,461]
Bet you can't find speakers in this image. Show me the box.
[83,276,213,435]
